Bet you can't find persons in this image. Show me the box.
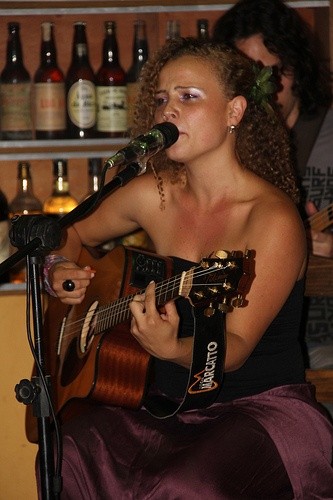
[34,35,333,500]
[211,1,332,371]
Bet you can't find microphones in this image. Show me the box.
[105,122,180,169]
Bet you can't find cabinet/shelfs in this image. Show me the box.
[0,0,333,292]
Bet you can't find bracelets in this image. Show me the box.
[41,254,70,304]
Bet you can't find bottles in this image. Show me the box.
[33,22,66,140]
[97,20,129,139]
[129,21,154,140]
[81,158,103,201]
[0,22,33,140]
[165,21,180,41]
[66,22,97,139]
[43,159,79,214]
[11,160,43,285]
[0,188,11,285]
[196,19,208,38]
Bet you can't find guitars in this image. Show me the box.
[25,246,252,445]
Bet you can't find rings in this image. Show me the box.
[62,279,75,292]
